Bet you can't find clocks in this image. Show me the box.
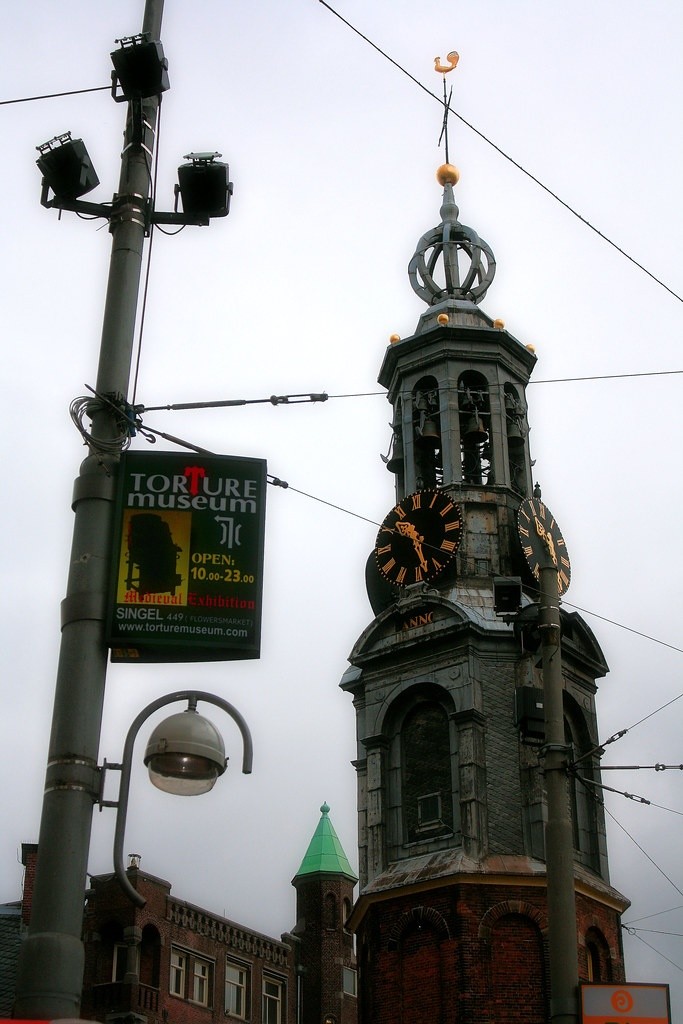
[374,490,464,588]
[516,497,571,597]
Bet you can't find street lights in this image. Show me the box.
[1,33,233,1024]
